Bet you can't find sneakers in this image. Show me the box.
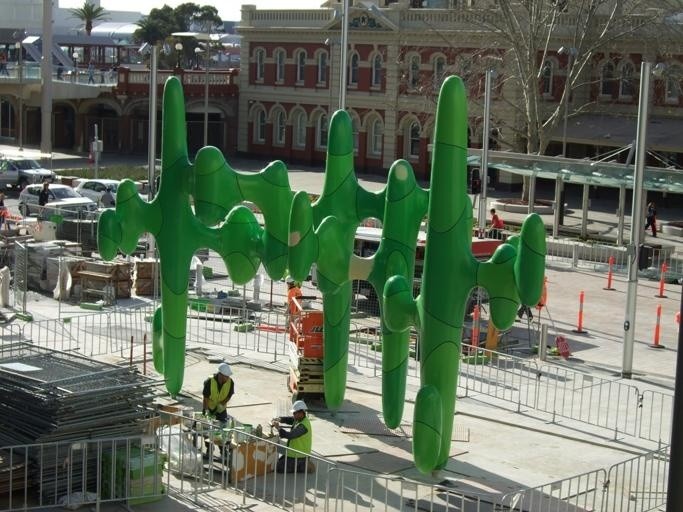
[307,461,315,473]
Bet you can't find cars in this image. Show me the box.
[0,158,149,257]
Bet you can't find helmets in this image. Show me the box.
[290,399,307,412]
[285,275,296,284]
[217,363,233,376]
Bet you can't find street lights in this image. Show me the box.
[16,42,20,65]
[73,52,78,81]
[195,47,200,69]
[175,43,183,67]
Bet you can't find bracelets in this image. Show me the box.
[224,400,226,403]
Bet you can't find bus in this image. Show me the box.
[312,227,503,317]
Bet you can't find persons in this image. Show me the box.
[0,52,9,76]
[644,199,655,236]
[517,305,532,320]
[284,275,301,342]
[101,188,114,208]
[489,208,503,239]
[270,400,315,474]
[86,61,95,84]
[0,193,5,225]
[55,59,64,81]
[38,181,49,214]
[202,363,234,458]
